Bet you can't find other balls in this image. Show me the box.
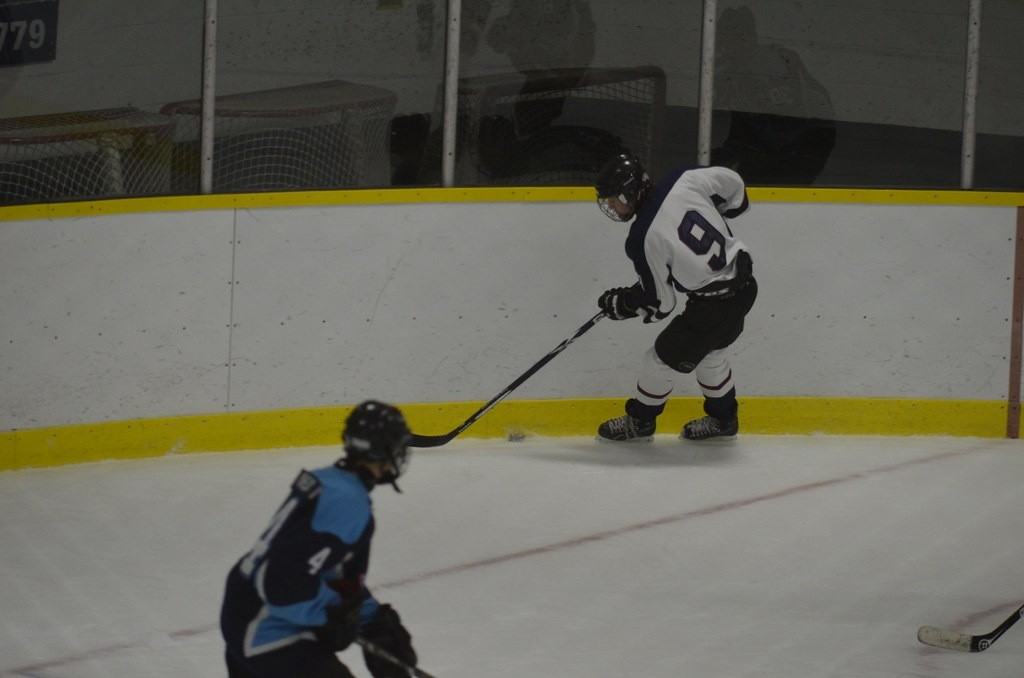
[509,433,526,443]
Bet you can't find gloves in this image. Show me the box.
[598,287,639,321]
[360,603,416,678]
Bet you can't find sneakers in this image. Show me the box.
[595,399,666,442]
[678,397,739,443]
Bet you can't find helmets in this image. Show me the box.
[342,402,409,485]
[595,153,654,222]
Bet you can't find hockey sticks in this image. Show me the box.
[353,633,442,678]
[916,602,1024,656]
[405,309,608,450]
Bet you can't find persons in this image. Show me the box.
[410,0,841,190]
[219,401,435,678]
[594,153,757,445]
